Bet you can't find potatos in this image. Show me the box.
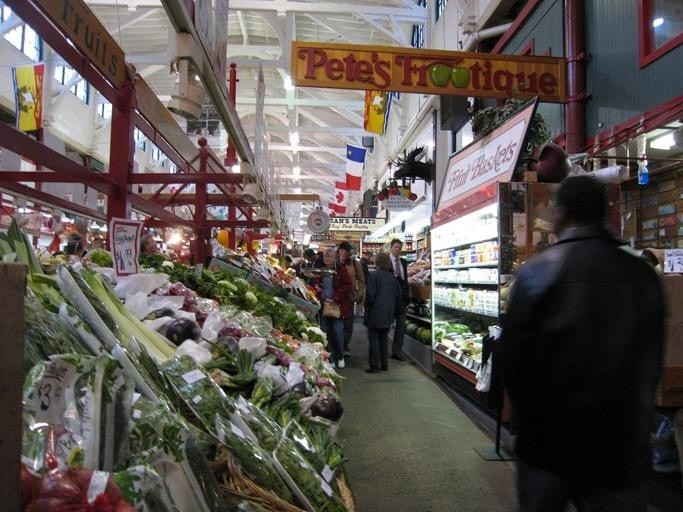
[19,458,135,512]
[151,282,337,392]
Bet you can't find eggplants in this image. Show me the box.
[289,379,315,405]
[314,391,343,422]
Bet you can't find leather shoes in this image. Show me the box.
[391,351,405,361]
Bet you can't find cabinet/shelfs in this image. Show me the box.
[309,160,681,452]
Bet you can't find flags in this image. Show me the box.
[346,144,366,191]
[363,90,392,136]
[12,64,44,131]
[328,180,348,213]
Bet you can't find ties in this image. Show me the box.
[395,259,401,277]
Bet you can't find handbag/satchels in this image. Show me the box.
[323,299,342,318]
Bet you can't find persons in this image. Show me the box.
[285,242,373,368]
[500,177,667,512]
[363,252,402,372]
[388,239,409,360]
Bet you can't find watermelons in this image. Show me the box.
[404,320,432,345]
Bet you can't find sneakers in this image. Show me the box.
[365,366,388,373]
[331,350,350,369]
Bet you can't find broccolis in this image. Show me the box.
[142,253,330,348]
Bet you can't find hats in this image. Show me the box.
[338,242,354,250]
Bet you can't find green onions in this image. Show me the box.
[34,263,181,367]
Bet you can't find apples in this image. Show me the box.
[429,64,450,87]
[450,68,470,88]
